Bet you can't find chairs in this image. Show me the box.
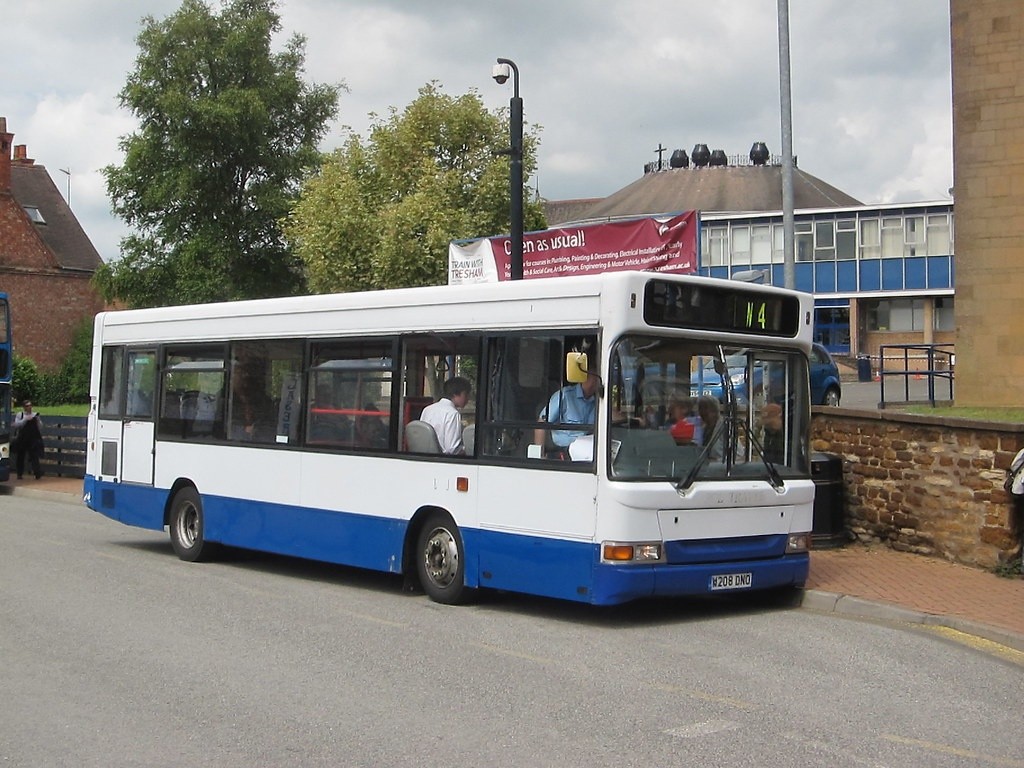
[110,386,476,458]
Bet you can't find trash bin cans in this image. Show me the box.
[806,453,849,550]
[857,357,872,381]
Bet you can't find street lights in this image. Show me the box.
[492,57,524,282]
[60,167,71,208]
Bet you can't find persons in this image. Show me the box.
[14,400,47,479]
[1010,447,1024,545]
[309,383,351,441]
[631,396,744,463]
[419,377,472,456]
[757,404,785,464]
[535,367,597,457]
[361,405,389,440]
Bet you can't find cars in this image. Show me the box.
[686,340,842,413]
[611,357,674,393]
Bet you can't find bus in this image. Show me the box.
[81,267,814,604]
[0,292,14,482]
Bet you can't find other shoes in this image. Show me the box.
[17,476,23,479]
[36,470,45,480]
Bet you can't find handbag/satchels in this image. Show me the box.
[1004,474,1015,493]
[9,436,17,453]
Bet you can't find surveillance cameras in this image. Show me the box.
[491,64,510,84]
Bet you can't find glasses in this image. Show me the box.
[24,404,32,407]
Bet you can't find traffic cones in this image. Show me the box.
[873,368,880,382]
[914,368,923,380]
